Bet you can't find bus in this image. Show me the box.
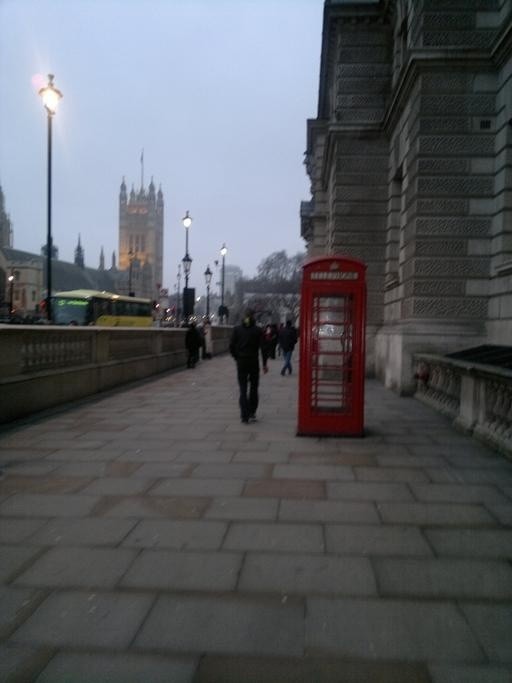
[50,288,153,326]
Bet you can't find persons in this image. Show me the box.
[11,313,80,326]
[258,316,298,377]
[185,321,210,368]
[226,311,270,422]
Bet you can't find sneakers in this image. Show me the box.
[262,367,294,376]
[240,417,256,424]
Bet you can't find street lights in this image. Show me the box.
[38,73,64,322]
[8,272,14,318]
[175,210,224,327]
[127,251,134,296]
